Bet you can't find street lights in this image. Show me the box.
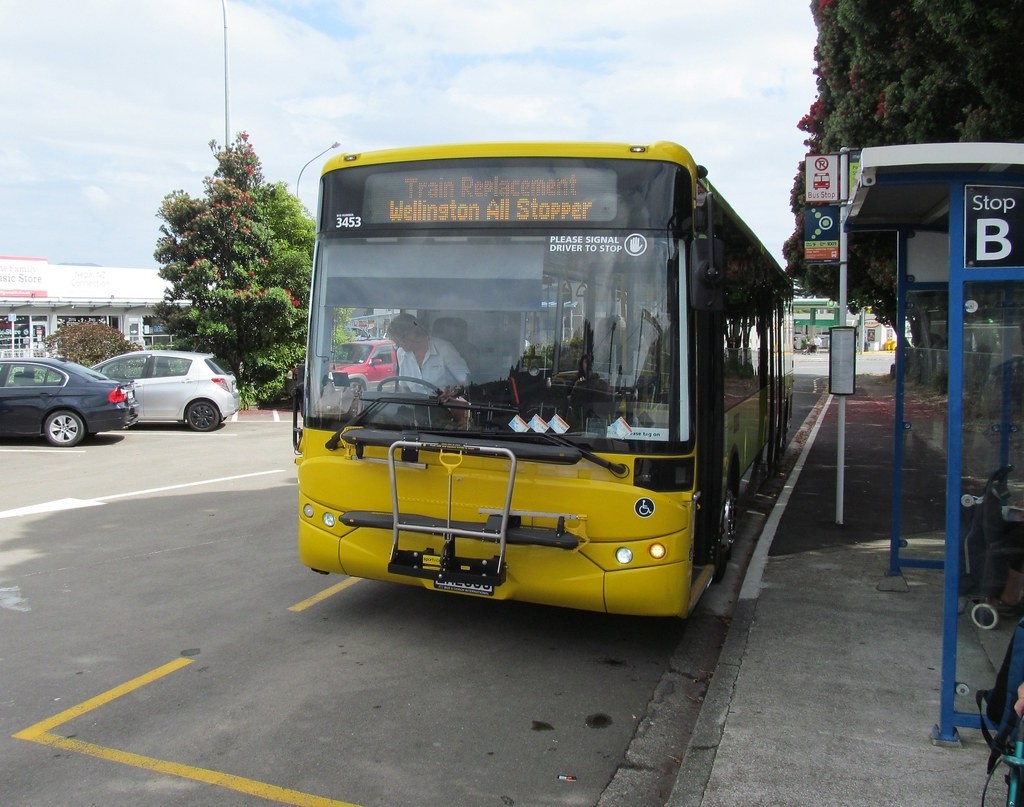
[297,142,341,197]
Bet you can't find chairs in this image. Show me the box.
[14,372,23,384]
[445,340,484,391]
[23,369,35,384]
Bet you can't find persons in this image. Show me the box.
[813,335,822,355]
[386,313,472,405]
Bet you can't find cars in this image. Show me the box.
[88,350,242,432]
[0,357,139,447]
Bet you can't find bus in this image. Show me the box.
[292,142,795,619]
